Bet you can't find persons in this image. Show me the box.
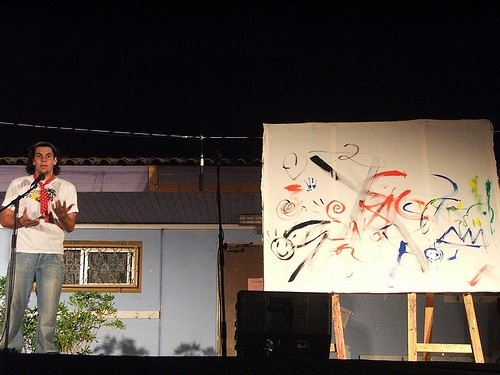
[0,143,79,354]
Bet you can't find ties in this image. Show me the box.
[33,172,56,222]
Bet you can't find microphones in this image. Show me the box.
[199,140,204,191]
[31,173,45,186]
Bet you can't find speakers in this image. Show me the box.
[234,290,332,358]
